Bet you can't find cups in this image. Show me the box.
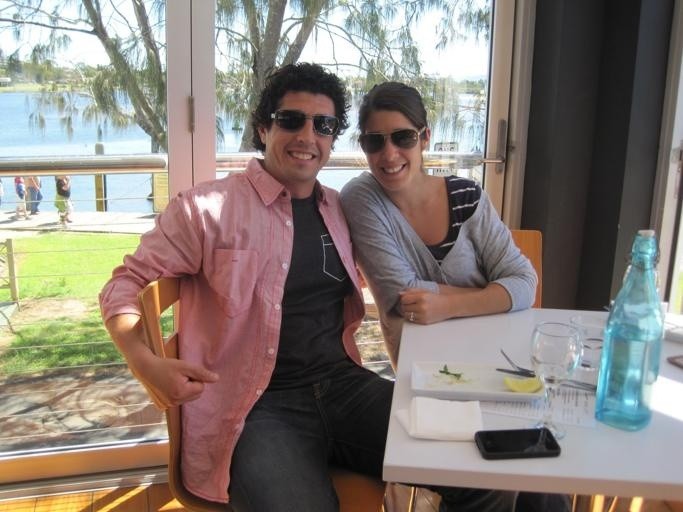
[581,336,603,370]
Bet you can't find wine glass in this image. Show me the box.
[530,322,584,444]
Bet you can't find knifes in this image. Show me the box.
[496,367,596,393]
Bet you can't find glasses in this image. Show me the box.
[359,126,426,153]
[271,110,340,136]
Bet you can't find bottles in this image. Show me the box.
[593,231,663,432]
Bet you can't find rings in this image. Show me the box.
[408,312,415,322]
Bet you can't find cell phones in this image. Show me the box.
[474,427,562,459]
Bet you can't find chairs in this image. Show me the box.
[133,276,386,511]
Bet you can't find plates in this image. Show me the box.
[409,359,550,405]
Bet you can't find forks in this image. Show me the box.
[500,349,596,388]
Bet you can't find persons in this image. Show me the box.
[13,176,32,221]
[334,79,579,511]
[53,174,72,226]
[26,175,44,215]
[99,60,525,511]
